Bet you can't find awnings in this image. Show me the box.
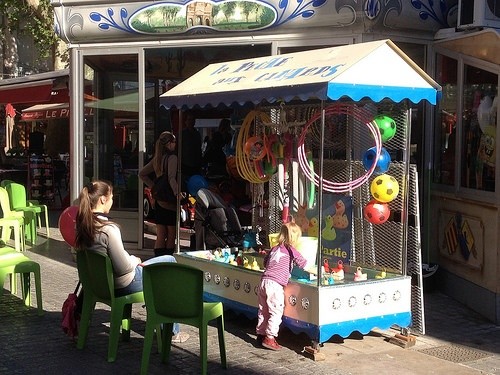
[0,68,95,122]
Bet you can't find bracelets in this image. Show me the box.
[174,192,177,196]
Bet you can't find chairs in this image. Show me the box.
[139,262,227,375]
[75,249,163,365]
[0,180,48,317]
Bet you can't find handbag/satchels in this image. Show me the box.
[62,280,81,341]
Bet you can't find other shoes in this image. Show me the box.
[258,335,262,345]
[171,332,190,342]
[261,335,282,351]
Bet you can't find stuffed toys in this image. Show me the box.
[477,126,496,170]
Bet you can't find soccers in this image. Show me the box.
[363,115,399,225]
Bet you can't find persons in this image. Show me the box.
[257,223,326,350]
[77,180,191,342]
[139,129,182,258]
